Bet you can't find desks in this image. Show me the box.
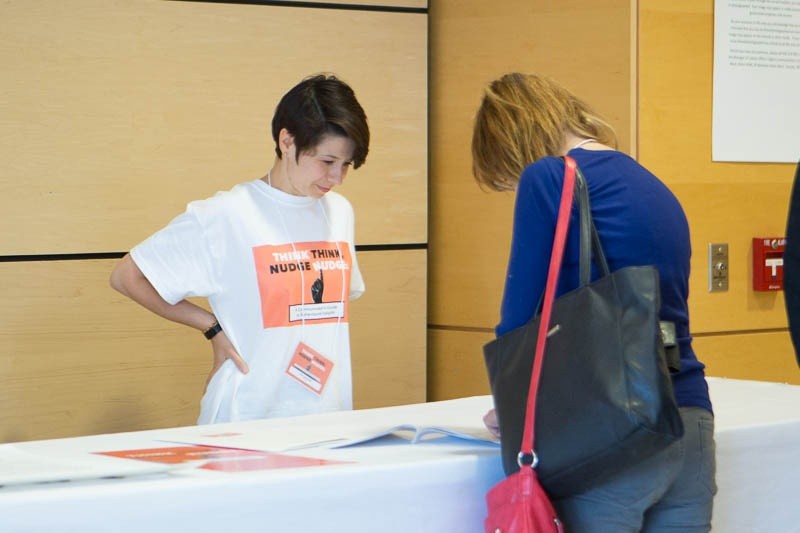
[0,376,800,533]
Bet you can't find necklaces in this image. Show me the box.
[575,138,597,149]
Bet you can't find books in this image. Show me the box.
[331,422,503,448]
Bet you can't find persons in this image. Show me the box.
[108,73,371,426]
[472,72,718,533]
[783,162,800,370]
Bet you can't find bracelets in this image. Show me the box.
[204,323,222,340]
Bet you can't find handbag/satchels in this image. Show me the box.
[484,464,563,533]
[483,159,685,502]
